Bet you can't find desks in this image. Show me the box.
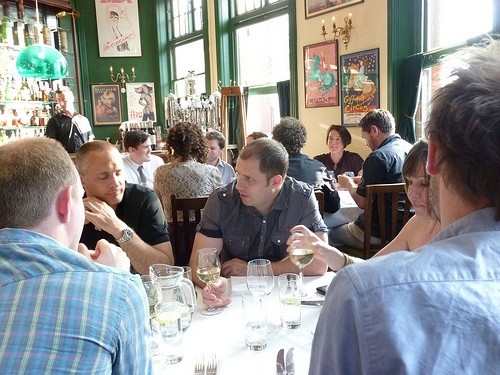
[151,270,339,375]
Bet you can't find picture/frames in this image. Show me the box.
[302,39,340,109]
[339,48,379,128]
[305,0,365,19]
[91,81,124,126]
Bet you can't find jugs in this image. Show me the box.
[148,263,197,335]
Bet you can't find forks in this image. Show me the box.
[194,356,218,375]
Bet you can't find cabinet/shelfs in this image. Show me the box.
[0,0,84,143]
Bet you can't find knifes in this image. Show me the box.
[277,348,294,374]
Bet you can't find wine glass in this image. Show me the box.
[289,228,315,298]
[196,248,224,316]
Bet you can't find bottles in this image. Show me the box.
[0,0,68,143]
[120,121,157,150]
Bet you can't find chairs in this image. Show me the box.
[363,183,409,259]
[171,193,207,268]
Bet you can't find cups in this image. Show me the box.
[278,273,303,329]
[246,259,274,295]
[242,289,267,351]
[346,172,354,178]
[328,171,335,180]
[141,266,193,364]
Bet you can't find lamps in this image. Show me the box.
[320,14,354,49]
[110,66,137,94]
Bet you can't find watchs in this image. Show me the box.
[117,228,134,244]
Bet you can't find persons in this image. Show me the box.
[272,110,414,249]
[45,87,94,154]
[286,142,442,271]
[121,119,268,260]
[188,139,330,307]
[76,140,174,276]
[308,50,500,375]
[0,137,154,375]
[96,90,117,117]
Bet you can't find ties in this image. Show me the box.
[137,166,147,187]
